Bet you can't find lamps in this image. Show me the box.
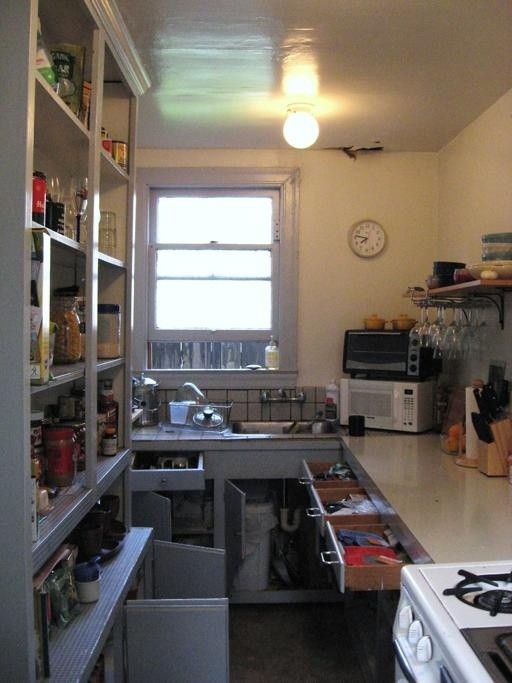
[283,102,319,149]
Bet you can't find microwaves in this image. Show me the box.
[337,378,436,432]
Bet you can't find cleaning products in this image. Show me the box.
[324,379,339,421]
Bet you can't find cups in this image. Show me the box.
[348,414,365,435]
[454,269,473,283]
[46,175,61,203]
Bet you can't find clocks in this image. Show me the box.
[347,219,386,258]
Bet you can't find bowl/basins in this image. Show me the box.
[426,261,466,285]
[363,317,388,329]
[390,317,418,329]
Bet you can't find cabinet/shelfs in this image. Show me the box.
[299,458,435,683]
[0,0,234,683]
[130,449,342,605]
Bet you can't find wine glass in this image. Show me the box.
[408,305,496,363]
[70,175,88,245]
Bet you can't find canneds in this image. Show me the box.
[30,410,44,448]
[46,427,73,487]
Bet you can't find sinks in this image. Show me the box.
[225,422,283,435]
[283,419,342,438]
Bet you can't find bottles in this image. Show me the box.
[325,378,340,423]
[98,302,121,358]
[32,169,47,226]
[102,427,118,456]
[264,334,281,368]
[51,294,82,361]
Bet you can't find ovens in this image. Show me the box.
[342,327,445,379]
[392,635,423,682]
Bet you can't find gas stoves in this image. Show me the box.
[392,560,511,682]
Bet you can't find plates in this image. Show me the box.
[467,232,511,280]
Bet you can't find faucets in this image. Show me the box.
[182,381,215,408]
[277,389,288,401]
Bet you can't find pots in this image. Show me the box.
[132,372,161,426]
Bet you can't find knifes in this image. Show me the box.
[470,359,510,443]
